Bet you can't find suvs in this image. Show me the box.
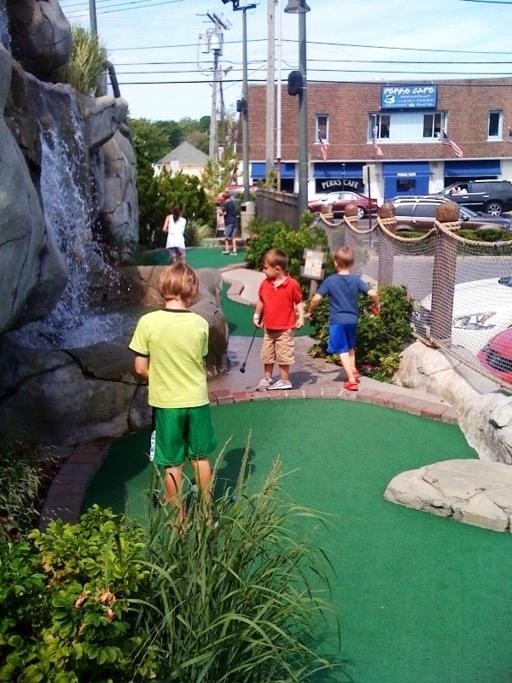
[418,178,512,216]
[375,194,511,232]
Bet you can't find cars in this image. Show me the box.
[476,324,512,397]
[308,190,378,219]
[408,266,512,356]
[212,184,261,204]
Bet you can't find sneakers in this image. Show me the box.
[222,249,237,256]
[343,370,360,390]
[256,377,293,389]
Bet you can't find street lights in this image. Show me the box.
[283,0,314,226]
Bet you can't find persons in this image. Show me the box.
[452,185,464,194]
[217,190,239,256]
[128,262,215,536]
[252,247,305,391]
[162,201,187,264]
[304,246,381,391]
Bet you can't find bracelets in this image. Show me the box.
[373,302,380,308]
[254,312,261,318]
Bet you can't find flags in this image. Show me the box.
[372,129,382,155]
[318,132,327,159]
[443,132,464,157]
